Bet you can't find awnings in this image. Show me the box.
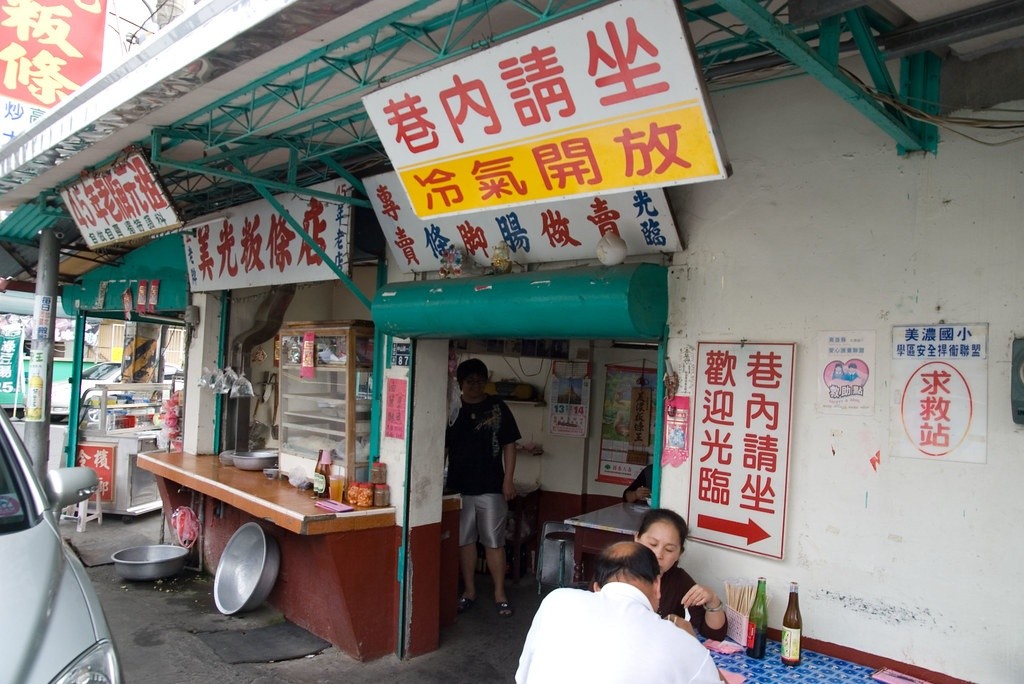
[0,200,84,249]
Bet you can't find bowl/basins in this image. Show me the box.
[252,448,278,463]
[263,468,279,480]
[213,523,280,614]
[230,451,278,471]
[110,545,189,579]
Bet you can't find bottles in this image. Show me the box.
[345,462,390,508]
[313,450,324,496]
[318,465,330,498]
[781,581,803,665]
[746,576,769,658]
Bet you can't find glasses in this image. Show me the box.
[466,378,485,384]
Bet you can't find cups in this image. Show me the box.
[321,450,332,465]
[329,476,345,503]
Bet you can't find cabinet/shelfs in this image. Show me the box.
[278,318,375,495]
[78,383,172,436]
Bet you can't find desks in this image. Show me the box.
[563,502,652,588]
[691,625,889,684]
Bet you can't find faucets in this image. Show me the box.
[177,485,186,493]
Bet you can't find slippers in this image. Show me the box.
[457,593,479,613]
[496,598,513,617]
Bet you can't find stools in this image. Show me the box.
[52,488,102,533]
[545,532,576,588]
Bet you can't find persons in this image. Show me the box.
[634,509,729,642]
[515,542,729,684]
[623,464,653,506]
[442,358,522,618]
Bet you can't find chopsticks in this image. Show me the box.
[724,583,757,617]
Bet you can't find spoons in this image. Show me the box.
[683,606,691,622]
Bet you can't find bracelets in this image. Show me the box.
[704,601,723,612]
